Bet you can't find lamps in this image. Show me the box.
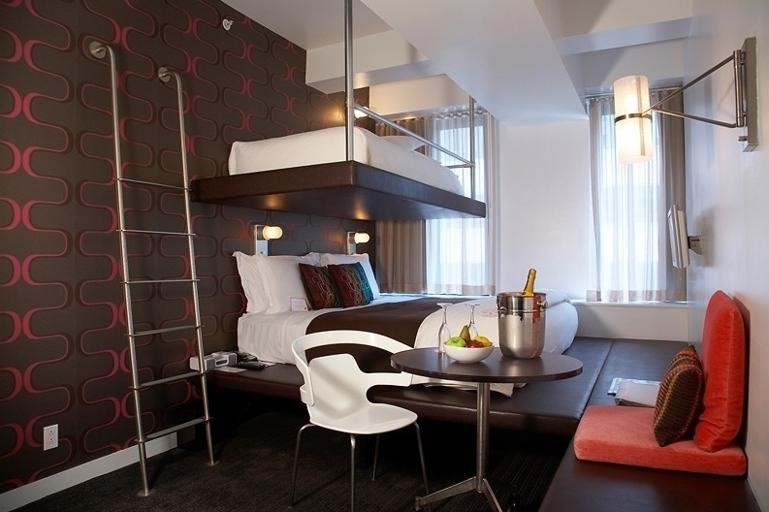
[611,36,760,164]
[252,224,284,254]
[347,232,371,254]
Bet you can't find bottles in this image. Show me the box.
[521,269,536,297]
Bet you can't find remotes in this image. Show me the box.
[237,360,265,370]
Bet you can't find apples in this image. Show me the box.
[446,336,464,346]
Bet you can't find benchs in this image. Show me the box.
[539,338,760,512]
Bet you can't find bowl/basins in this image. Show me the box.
[443,344,494,364]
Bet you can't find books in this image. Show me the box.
[615,379,657,409]
[606,375,661,396]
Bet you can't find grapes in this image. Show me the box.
[468,339,483,348]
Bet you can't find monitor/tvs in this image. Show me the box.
[667,204,690,270]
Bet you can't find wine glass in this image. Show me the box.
[466,302,481,338]
[436,302,451,352]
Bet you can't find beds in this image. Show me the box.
[228,125,465,195]
[233,252,578,400]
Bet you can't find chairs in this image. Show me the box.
[287,329,430,512]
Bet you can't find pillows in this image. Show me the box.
[331,266,373,309]
[323,253,381,298]
[379,133,424,151]
[696,289,745,453]
[655,345,702,447]
[300,264,345,310]
[254,253,323,314]
[235,251,319,313]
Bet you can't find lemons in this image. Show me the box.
[475,335,491,346]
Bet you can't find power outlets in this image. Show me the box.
[42,425,58,451]
